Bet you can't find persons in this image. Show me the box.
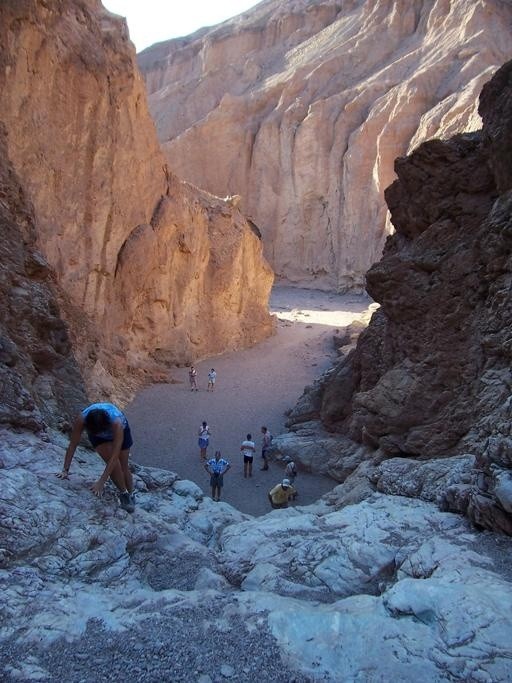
[198,421,211,463]
[56,400,136,515]
[259,425,273,470]
[267,476,298,508]
[286,453,298,501]
[188,365,198,392]
[206,368,217,394]
[204,451,231,502]
[240,433,253,479]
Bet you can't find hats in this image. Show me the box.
[282,478,290,488]
[282,455,291,462]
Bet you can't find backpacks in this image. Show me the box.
[289,463,297,477]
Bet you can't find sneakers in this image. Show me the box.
[207,388,214,392]
[260,465,269,471]
[200,456,209,463]
[191,388,198,392]
[119,490,136,506]
[244,471,252,478]
[212,497,221,502]
